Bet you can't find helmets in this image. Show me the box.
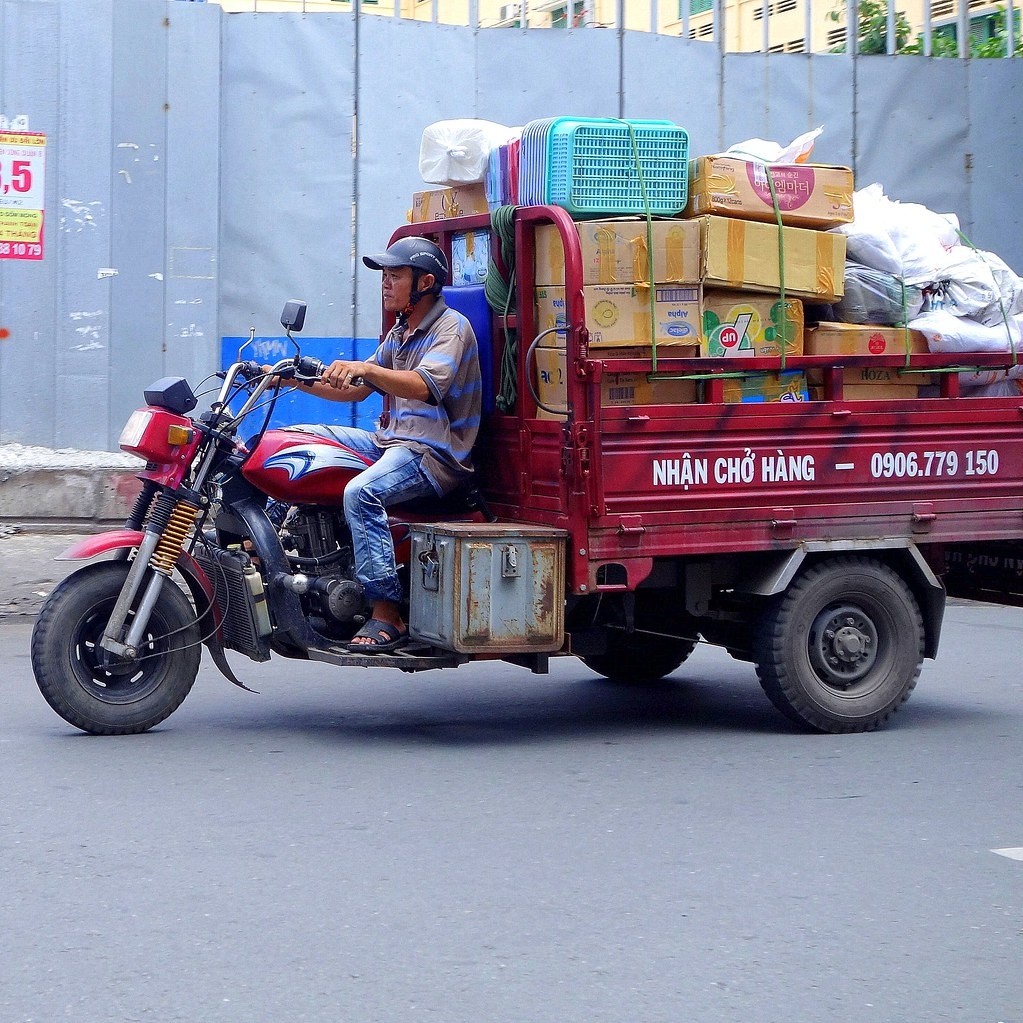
[363,236,449,290]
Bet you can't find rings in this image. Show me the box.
[347,374,353,379]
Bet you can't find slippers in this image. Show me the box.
[347,619,409,651]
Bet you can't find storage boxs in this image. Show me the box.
[407,116,931,400]
[401,522,570,655]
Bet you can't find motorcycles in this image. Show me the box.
[30,206,1022,734]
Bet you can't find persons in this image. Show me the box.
[251,236,482,652]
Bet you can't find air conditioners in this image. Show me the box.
[499,4,518,21]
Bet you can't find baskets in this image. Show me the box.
[485,117,690,218]
